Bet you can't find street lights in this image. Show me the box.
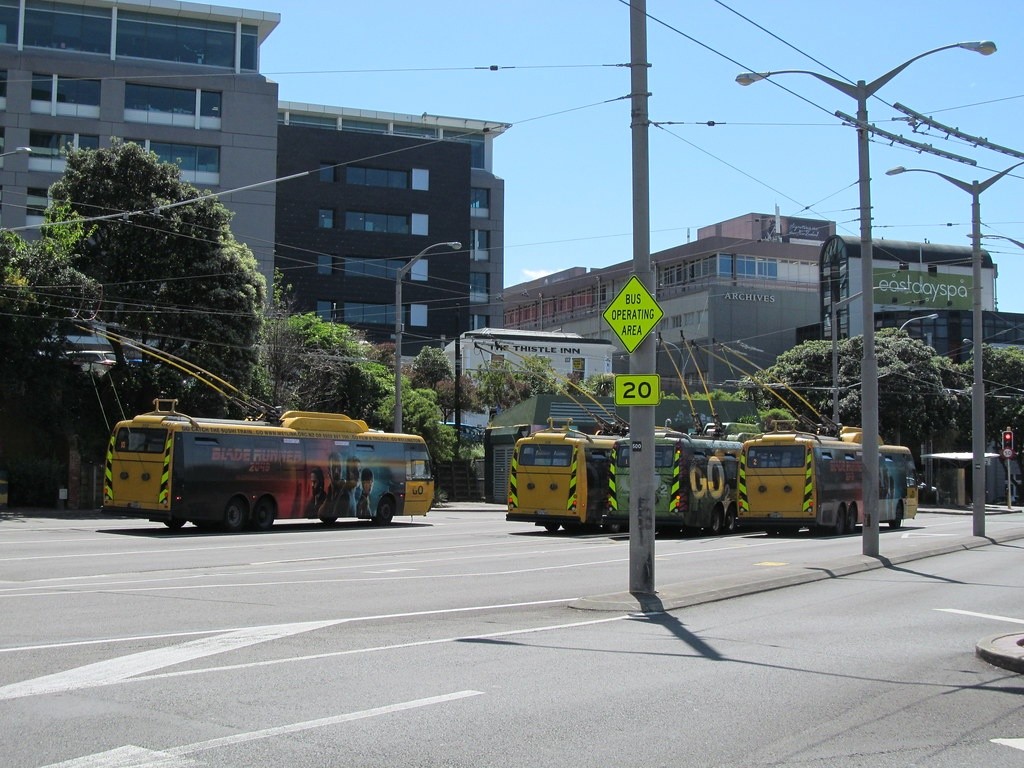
[736,41,996,554]
[832,285,910,428]
[655,339,684,400]
[394,240,461,435]
[885,161,1024,537]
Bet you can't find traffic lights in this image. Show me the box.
[1003,432,1013,450]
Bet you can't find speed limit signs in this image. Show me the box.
[1002,448,1014,460]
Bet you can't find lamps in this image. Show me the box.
[948,301,952,306]
[892,297,897,304]
[919,300,925,304]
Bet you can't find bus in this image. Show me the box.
[602,422,772,535]
[101,398,434,532]
[735,426,924,535]
[506,416,672,534]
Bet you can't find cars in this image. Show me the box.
[65,350,117,378]
[124,357,154,370]
[906,476,937,502]
